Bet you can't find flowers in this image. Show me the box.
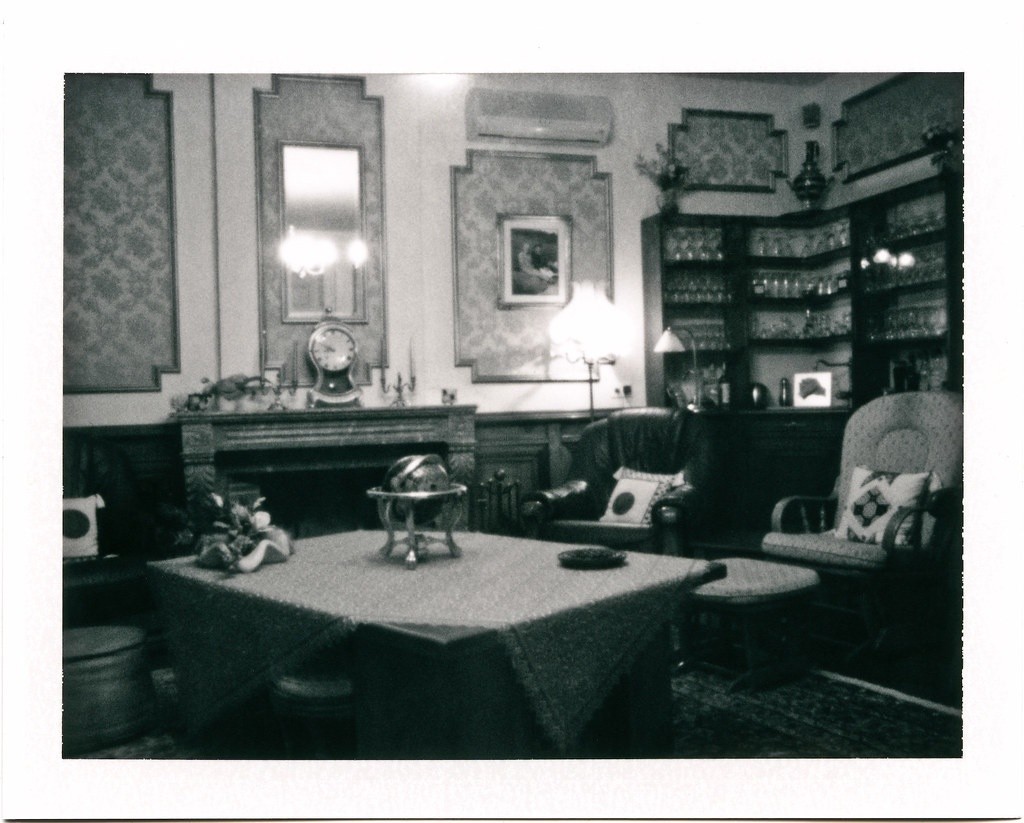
[632,143,691,182]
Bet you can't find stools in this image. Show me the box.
[267,660,357,760]
[63,626,150,749]
[683,557,819,696]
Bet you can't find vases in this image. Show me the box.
[653,175,685,215]
[790,141,827,209]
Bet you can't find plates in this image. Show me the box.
[558,549,627,566]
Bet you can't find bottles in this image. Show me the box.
[892,352,942,391]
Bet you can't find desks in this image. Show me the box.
[141,528,728,760]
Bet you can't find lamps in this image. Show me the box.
[652,324,703,412]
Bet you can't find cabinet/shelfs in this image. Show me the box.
[637,179,963,503]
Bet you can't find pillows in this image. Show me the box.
[600,464,687,529]
[64,492,102,558]
[830,465,934,545]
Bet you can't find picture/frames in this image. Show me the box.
[493,211,574,312]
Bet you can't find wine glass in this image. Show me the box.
[661,204,950,344]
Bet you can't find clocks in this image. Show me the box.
[305,306,368,409]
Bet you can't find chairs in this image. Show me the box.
[62,420,184,629]
[516,405,718,556]
[759,388,964,658]
